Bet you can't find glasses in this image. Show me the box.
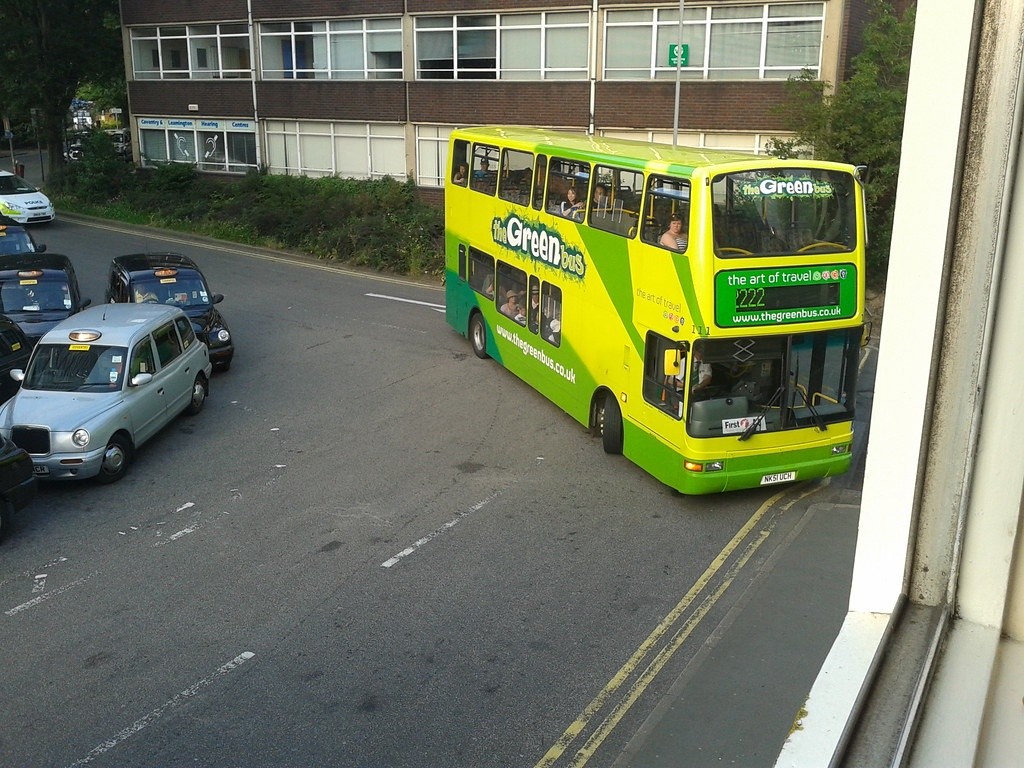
[670,213,684,219]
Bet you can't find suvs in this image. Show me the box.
[1,315,36,407]
[104,253,235,373]
[0,251,93,352]
[0,303,214,485]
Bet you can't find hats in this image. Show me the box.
[530,286,539,294]
[480,158,489,165]
[506,290,518,300]
[550,319,561,332]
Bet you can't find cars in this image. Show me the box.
[0,433,42,542]
[0,224,48,261]
[67,106,133,163]
[0,169,56,229]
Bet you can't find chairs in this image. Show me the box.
[85,348,125,384]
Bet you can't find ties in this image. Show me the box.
[692,361,701,388]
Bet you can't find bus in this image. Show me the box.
[445,126,875,496]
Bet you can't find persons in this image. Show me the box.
[474,161,490,181]
[486,281,560,344]
[659,211,688,253]
[135,284,158,304]
[453,163,467,184]
[667,348,713,401]
[560,183,617,221]
